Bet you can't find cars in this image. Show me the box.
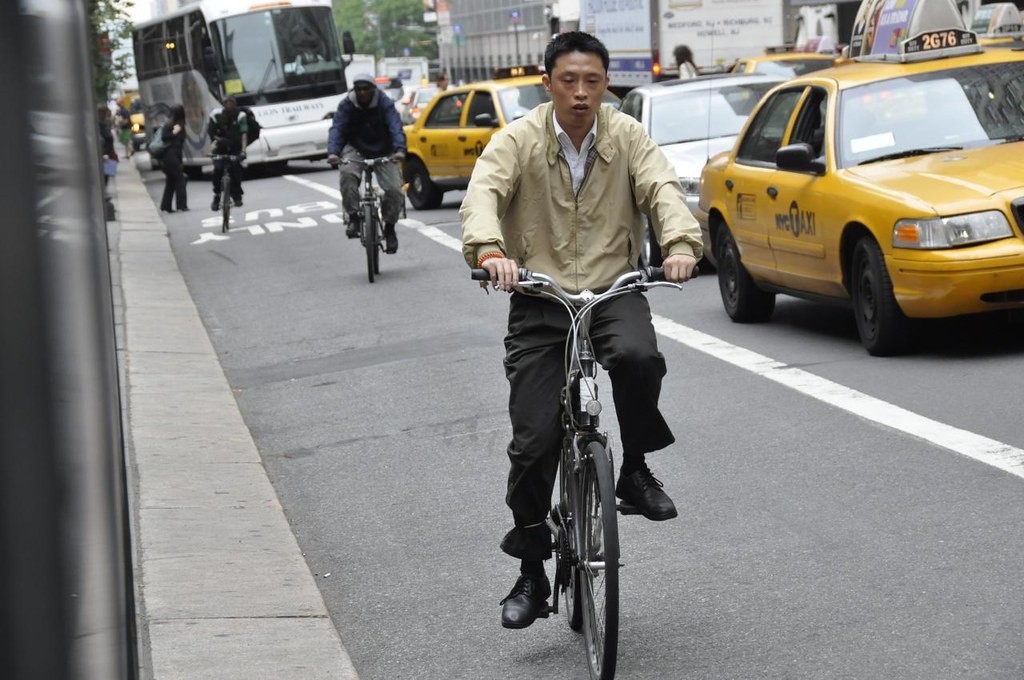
[615,73,827,274]
[696,0,1024,358]
[399,65,624,212]
[109,92,146,153]
[341,0,1024,128]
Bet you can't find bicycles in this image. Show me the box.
[207,152,249,233]
[468,260,701,680]
[328,154,406,283]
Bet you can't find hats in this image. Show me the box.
[222,95,236,104]
[353,72,377,88]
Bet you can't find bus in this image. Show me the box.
[128,1,356,180]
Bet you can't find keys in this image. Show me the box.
[480,282,491,295]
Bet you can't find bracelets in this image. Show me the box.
[477,252,504,269]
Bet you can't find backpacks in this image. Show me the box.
[237,108,261,147]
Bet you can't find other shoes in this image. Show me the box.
[161,207,176,213]
[384,228,398,251]
[346,218,360,236]
[211,195,220,211]
[232,193,242,206]
[177,205,189,211]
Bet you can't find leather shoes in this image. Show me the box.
[500,574,551,629]
[615,463,678,521]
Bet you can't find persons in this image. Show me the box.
[116,99,134,160]
[672,44,700,79]
[327,74,405,254]
[149,105,189,213]
[433,74,452,93]
[296,43,324,65]
[94,104,119,221]
[208,98,261,211]
[460,31,704,629]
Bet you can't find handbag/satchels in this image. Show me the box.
[148,121,174,153]
